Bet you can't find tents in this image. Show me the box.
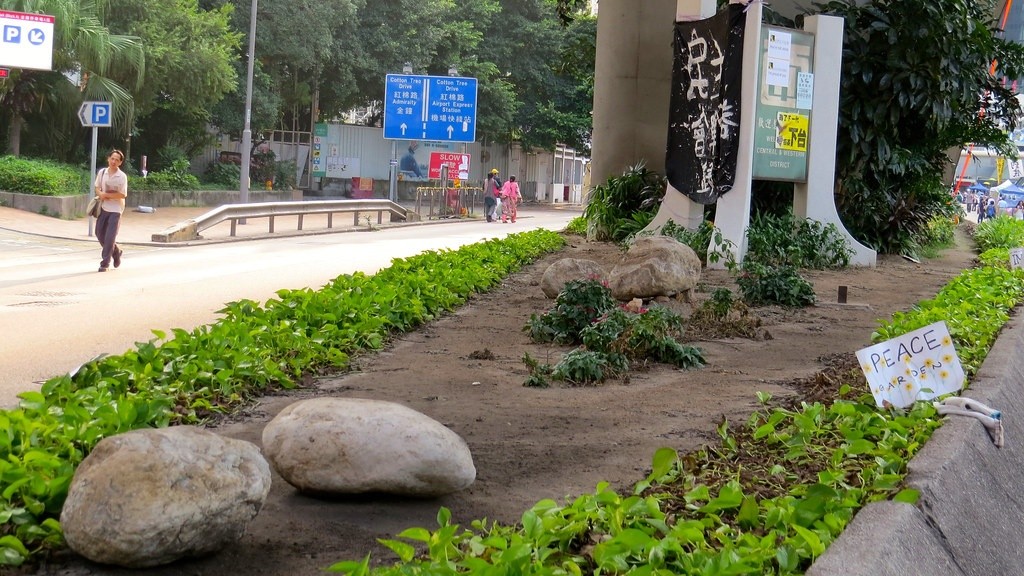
[969,183,989,194]
[990,180,1024,213]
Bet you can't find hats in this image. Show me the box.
[491,169,499,174]
[408,146,415,154]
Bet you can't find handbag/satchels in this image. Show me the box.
[492,184,500,196]
[86,196,102,218]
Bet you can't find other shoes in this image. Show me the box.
[488,215,493,220]
[113,250,123,268]
[511,221,517,223]
[503,221,508,223]
[487,219,491,222]
[98,265,111,272]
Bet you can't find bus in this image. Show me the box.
[952,147,1024,197]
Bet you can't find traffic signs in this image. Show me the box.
[384,74,477,145]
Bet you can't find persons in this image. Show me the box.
[958,190,1024,224]
[94,150,128,272]
[483,172,500,222]
[500,175,523,223]
[769,62,773,69]
[770,35,776,41]
[491,169,503,221]
